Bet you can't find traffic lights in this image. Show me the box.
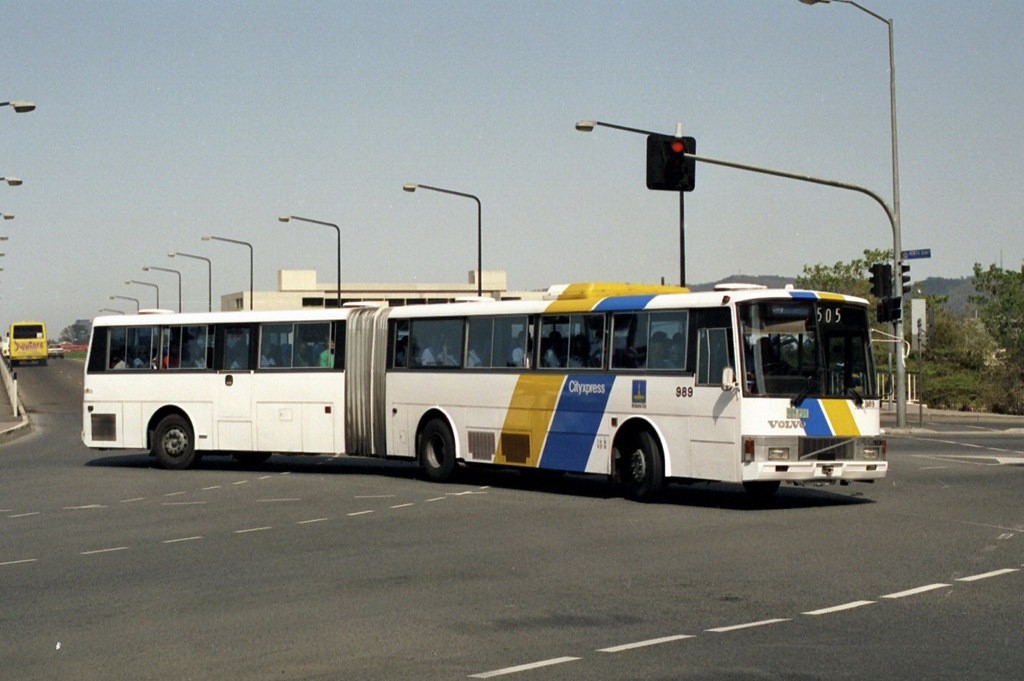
[867,264,883,298]
[881,296,902,322]
[901,265,911,295]
[646,132,696,192]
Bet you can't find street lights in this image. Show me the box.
[799,1,905,431]
[167,252,211,313]
[201,236,253,310]
[141,266,182,314]
[109,295,139,311]
[277,214,341,308]
[572,119,687,290]
[125,280,160,310]
[402,183,482,297]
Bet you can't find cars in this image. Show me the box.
[0,337,10,359]
[48,343,64,359]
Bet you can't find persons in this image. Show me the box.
[294,342,310,366]
[318,336,335,368]
[590,328,609,361]
[397,335,420,367]
[192,343,204,368]
[663,334,685,369]
[274,343,291,368]
[569,330,600,367]
[225,340,248,369]
[262,336,277,368]
[114,343,150,368]
[545,329,567,366]
[638,331,668,369]
[749,338,793,375]
[414,332,533,367]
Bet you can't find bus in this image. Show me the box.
[82,281,912,503]
[6,320,48,367]
